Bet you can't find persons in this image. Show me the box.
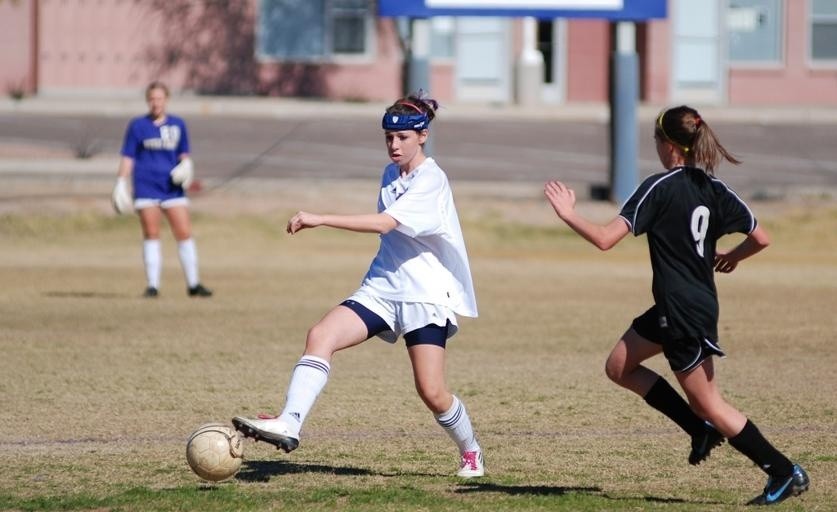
[112,81,212,296]
[544,106,810,504]
[232,94,485,478]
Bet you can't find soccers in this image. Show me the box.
[186,422,245,482]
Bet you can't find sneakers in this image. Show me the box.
[746,463,809,505]
[455,448,485,477]
[231,417,300,453]
[145,286,158,296]
[688,426,725,466]
[187,283,213,297]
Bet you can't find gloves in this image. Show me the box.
[110,177,129,214]
[170,158,194,190]
